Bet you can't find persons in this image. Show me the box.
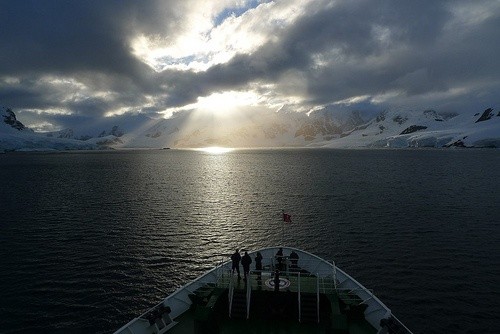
[275,248,283,262]
[377,318,388,334]
[231,249,241,274]
[255,252,262,280]
[241,251,251,281]
[289,250,299,264]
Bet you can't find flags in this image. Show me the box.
[284,214,292,222]
